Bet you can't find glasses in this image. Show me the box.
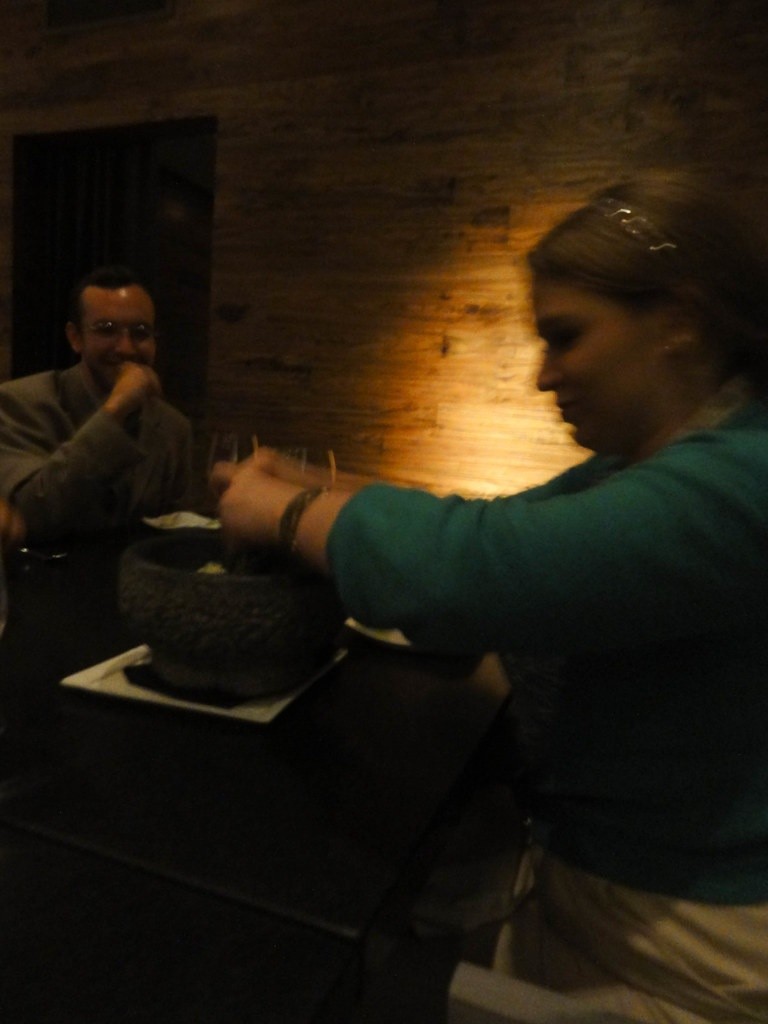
[83,318,157,343]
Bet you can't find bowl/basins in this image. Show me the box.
[115,527,348,701]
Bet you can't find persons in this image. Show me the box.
[212,169,768,1024]
[0,498,26,543]
[0,268,212,539]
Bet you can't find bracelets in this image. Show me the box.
[278,485,328,549]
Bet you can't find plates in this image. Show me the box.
[343,617,469,652]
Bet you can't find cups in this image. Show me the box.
[271,444,337,492]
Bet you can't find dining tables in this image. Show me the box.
[0,493,517,1024]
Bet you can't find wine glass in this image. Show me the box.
[207,432,237,518]
